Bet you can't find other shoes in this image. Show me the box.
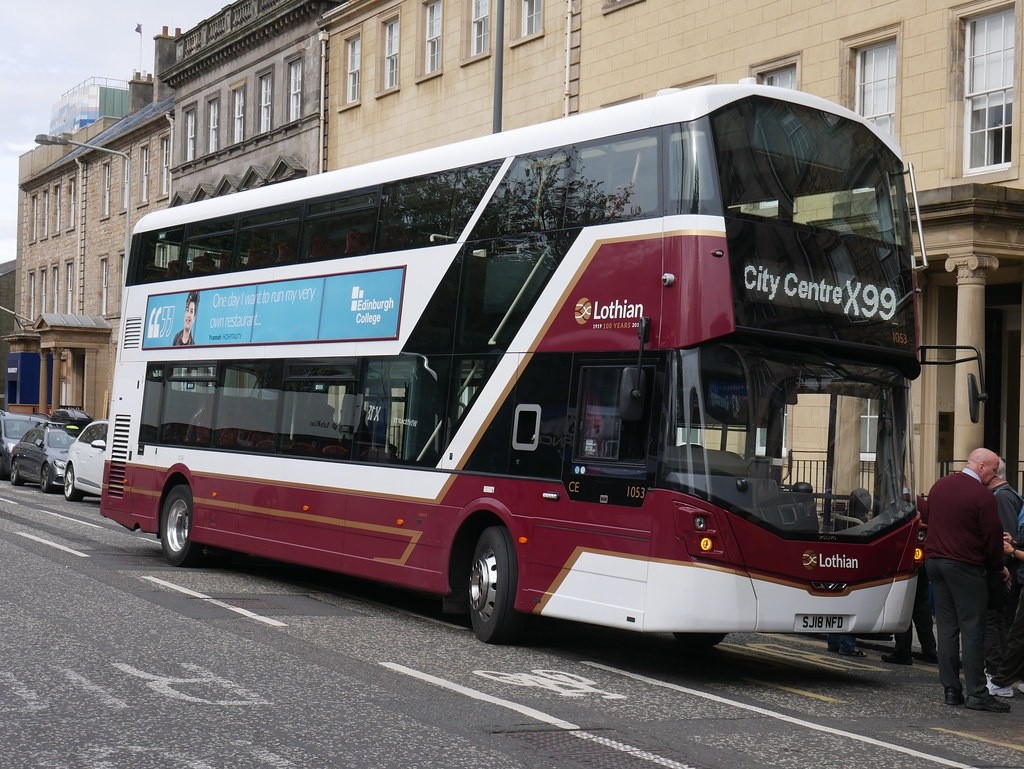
[838,648,868,657]
[827,645,838,652]
[913,654,938,665]
[881,654,913,665]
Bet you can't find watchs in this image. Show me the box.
[1010,549,1018,557]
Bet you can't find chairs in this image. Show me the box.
[140,223,417,282]
[144,403,390,459]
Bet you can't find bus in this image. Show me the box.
[100,76,989,648]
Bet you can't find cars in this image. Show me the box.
[9,421,83,494]
[0,409,53,480]
[63,420,109,502]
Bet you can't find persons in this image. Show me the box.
[173,292,199,346]
[820,447,1024,714]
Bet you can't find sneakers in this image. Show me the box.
[966,694,1012,712]
[986,681,1014,697]
[945,688,965,705]
